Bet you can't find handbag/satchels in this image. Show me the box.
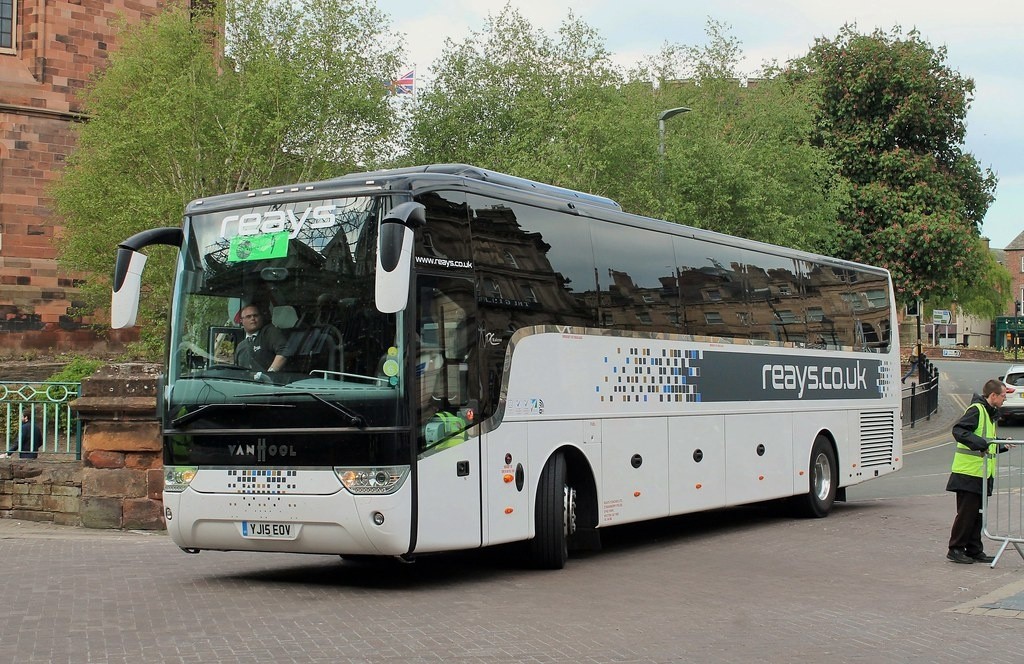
[909,355,918,363]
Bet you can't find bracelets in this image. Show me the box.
[268,368,279,372]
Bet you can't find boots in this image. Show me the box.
[901,377,905,384]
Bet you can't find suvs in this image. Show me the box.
[994,363,1024,426]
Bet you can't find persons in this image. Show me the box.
[234,304,290,372]
[901,340,929,384]
[946,379,1017,564]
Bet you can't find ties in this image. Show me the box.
[247,335,255,343]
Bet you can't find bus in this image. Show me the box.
[110,162,904,573]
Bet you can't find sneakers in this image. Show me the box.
[968,555,995,563]
[947,549,973,564]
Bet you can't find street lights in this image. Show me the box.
[658,106,692,183]
